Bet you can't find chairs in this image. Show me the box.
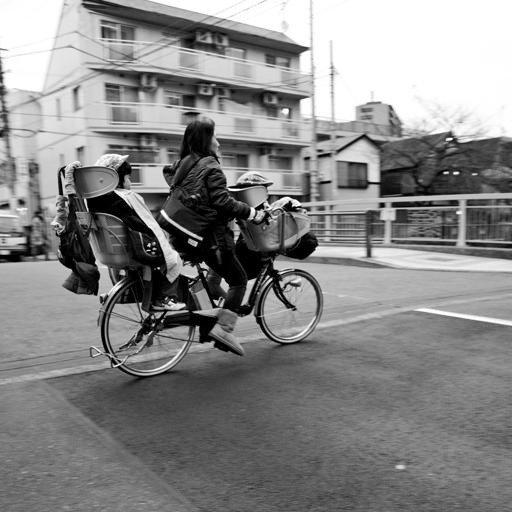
[58,165,167,315]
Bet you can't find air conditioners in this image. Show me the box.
[193,26,230,47]
[141,71,158,89]
[264,94,279,106]
[197,83,215,97]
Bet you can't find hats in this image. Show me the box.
[95,153,129,170]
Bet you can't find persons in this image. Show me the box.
[161,116,272,358]
[91,152,189,313]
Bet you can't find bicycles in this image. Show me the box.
[91,164,326,379]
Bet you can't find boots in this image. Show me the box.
[204,275,227,300]
[207,309,245,356]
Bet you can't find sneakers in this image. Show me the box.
[151,296,186,312]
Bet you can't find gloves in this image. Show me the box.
[247,207,269,225]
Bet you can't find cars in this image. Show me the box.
[0,209,28,261]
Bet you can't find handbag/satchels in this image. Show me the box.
[155,154,213,258]
